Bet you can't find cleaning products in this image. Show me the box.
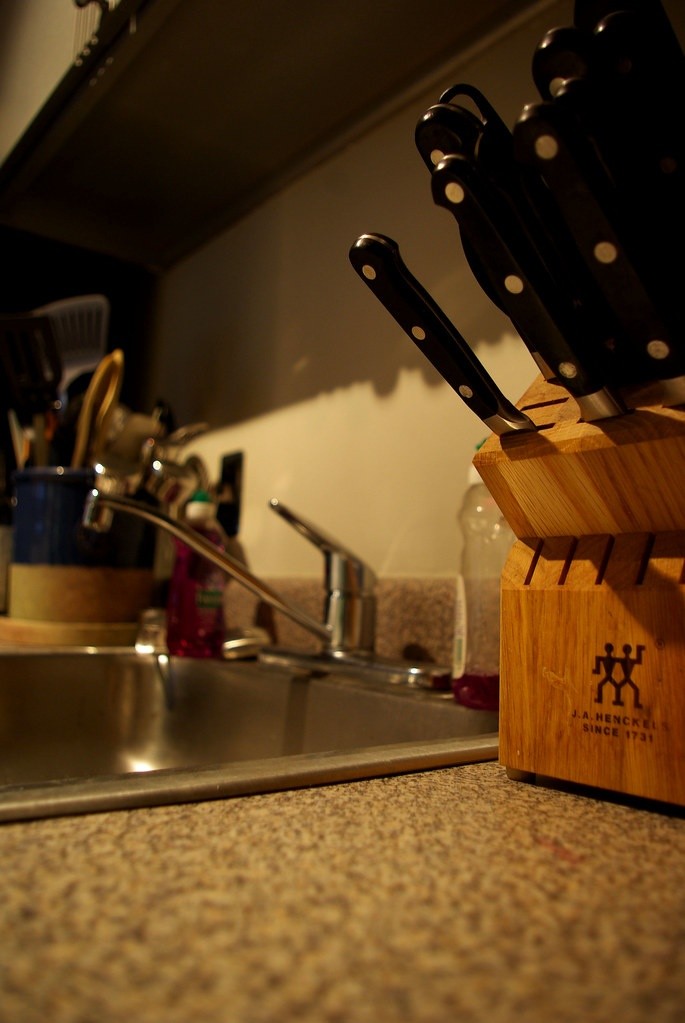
[450,439,520,713]
[165,488,231,659]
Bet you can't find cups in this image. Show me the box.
[11,468,162,626]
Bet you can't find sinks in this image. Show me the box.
[0,646,500,822]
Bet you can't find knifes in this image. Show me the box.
[418,0,685,422]
[349,233,536,435]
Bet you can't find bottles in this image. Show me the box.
[165,490,226,660]
[450,438,516,710]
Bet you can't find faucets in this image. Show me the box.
[81,487,379,666]
